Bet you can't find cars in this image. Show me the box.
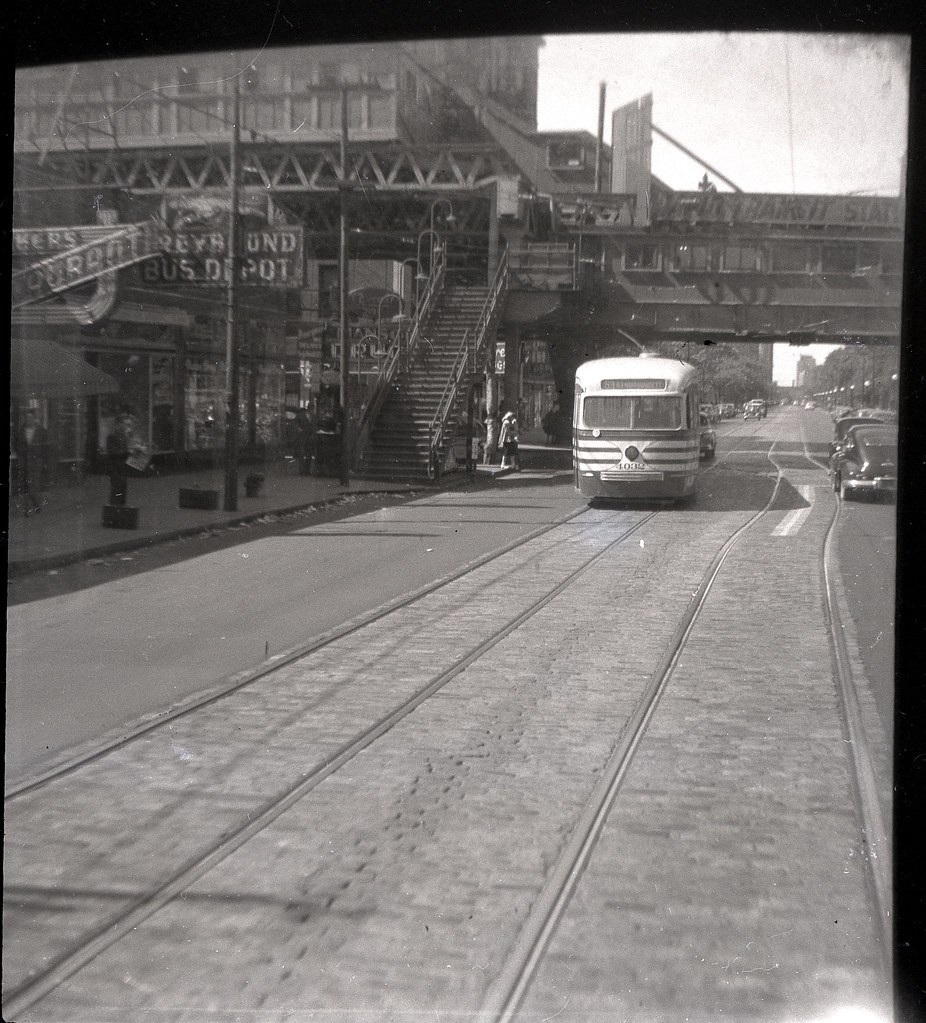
[829,418,886,461]
[699,414,717,459]
[831,407,896,424]
[828,424,897,502]
[698,402,736,423]
[793,398,817,410]
[742,403,761,421]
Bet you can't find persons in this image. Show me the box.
[295,398,344,480]
[541,400,563,445]
[106,415,135,504]
[482,410,521,470]
[16,410,55,516]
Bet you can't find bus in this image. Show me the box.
[573,353,700,506]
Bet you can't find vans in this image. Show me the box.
[749,399,767,418]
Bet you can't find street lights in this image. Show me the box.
[840,387,845,407]
[850,386,854,407]
[813,389,833,410]
[221,63,259,513]
[306,66,385,488]
[834,390,837,408]
[892,373,899,412]
[864,381,871,408]
